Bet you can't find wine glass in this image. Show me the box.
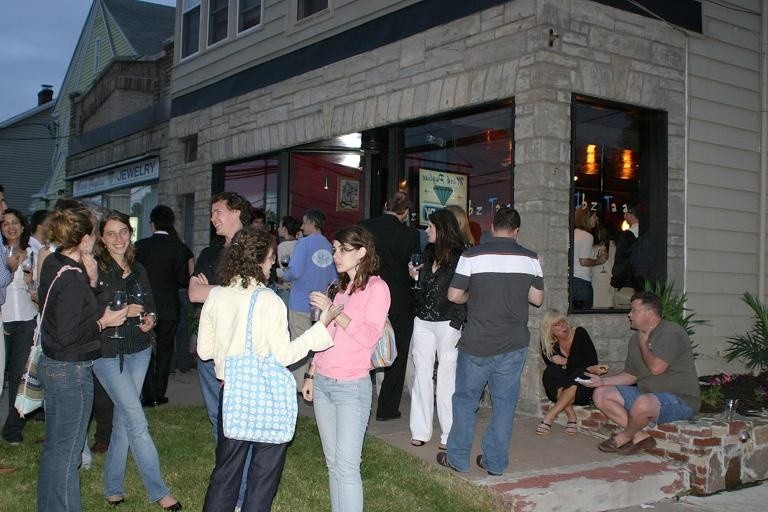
[132,283,144,326]
[20,256,35,291]
[598,248,608,273]
[6,245,18,278]
[281,255,290,272]
[411,253,422,289]
[108,291,127,338]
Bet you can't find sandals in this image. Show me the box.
[535,421,551,436]
[565,422,577,436]
[439,442,448,450]
[411,440,426,446]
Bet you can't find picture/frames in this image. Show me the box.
[336,176,361,213]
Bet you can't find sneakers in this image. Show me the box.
[476,455,503,477]
[437,452,460,473]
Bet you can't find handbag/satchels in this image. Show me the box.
[222,354,299,444]
[13,345,44,419]
[369,317,398,372]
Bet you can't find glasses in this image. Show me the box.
[552,319,564,326]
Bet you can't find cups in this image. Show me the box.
[310,291,322,321]
[725,399,736,421]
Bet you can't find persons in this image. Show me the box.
[573,200,650,310]
[187,189,421,512]
[408,205,474,449]
[534,308,610,437]
[2,191,196,511]
[437,206,545,476]
[574,288,702,456]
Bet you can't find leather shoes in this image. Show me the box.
[376,411,401,421]
[598,437,633,453]
[156,500,182,512]
[1,396,168,476]
[615,436,657,456]
[105,496,124,506]
